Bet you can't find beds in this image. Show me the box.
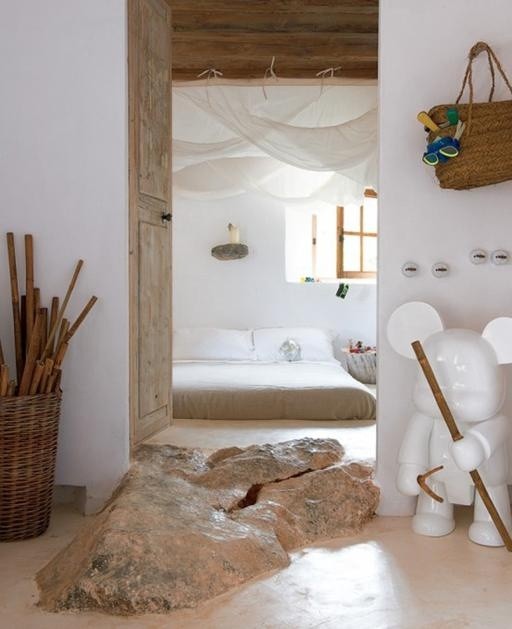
[173,327,373,421]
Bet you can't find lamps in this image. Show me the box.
[211,221,251,263]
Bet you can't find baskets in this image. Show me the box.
[1,389,63,543]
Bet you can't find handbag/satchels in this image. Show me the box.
[423,42,512,191]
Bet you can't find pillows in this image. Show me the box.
[175,327,331,365]
